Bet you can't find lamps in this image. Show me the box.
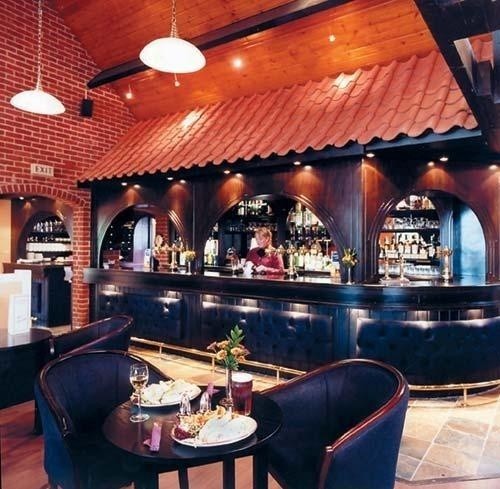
[134,0,208,88]
[8,0,65,117]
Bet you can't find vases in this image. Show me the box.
[345,266,353,286]
[187,259,192,275]
[153,252,171,269]
[219,365,233,407]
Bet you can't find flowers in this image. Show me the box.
[154,236,171,253]
[343,247,359,267]
[208,323,252,372]
[184,250,195,261]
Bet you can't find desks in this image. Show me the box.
[0,327,53,437]
[103,380,283,489]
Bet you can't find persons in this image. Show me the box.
[245,227,284,275]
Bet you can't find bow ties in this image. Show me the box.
[257,249,265,257]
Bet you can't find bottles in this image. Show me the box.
[199,391,211,412]
[414,195,436,209]
[276,219,332,272]
[179,390,192,416]
[379,232,440,259]
[203,238,218,265]
[25,218,72,243]
[238,200,266,215]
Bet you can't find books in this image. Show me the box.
[0,269,32,335]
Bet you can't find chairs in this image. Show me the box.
[251,358,409,489]
[33,349,189,489]
[33,311,137,435]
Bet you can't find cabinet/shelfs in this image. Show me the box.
[100,208,135,264]
[205,200,278,268]
[374,198,440,276]
[27,215,72,264]
[284,203,340,275]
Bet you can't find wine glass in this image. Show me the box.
[129,361,150,423]
[247,221,278,231]
[380,214,440,230]
[132,421,152,456]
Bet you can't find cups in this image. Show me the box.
[230,371,253,415]
[232,270,239,279]
[378,262,439,276]
[231,258,240,270]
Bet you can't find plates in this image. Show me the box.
[129,380,202,408]
[166,435,257,460]
[168,410,258,447]
